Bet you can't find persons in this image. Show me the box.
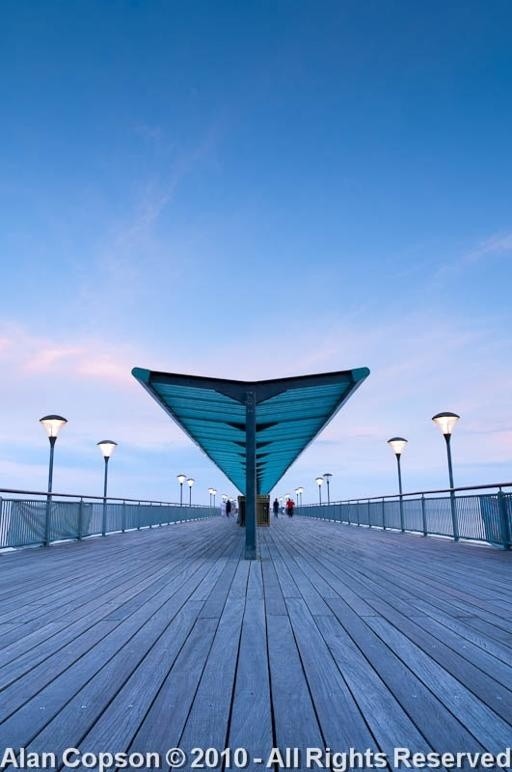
[221,499,237,518]
[285,497,295,518]
[273,497,278,519]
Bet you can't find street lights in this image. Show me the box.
[178,474,193,523]
[206,487,234,510]
[387,437,408,532]
[315,473,333,522]
[39,414,67,546]
[279,494,290,511]
[96,441,117,538]
[295,486,304,515]
[433,412,460,541]
